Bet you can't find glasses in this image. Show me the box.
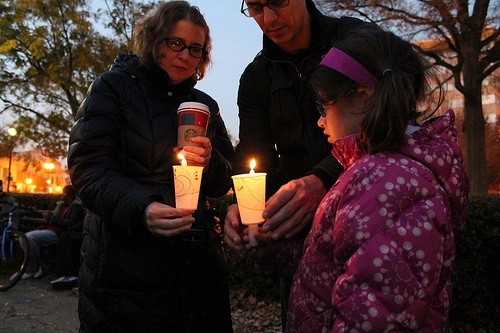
[165,38,205,58]
[314,89,357,117]
[241,0,290,17]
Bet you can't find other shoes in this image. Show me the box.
[34,265,42,278]
[21,272,33,278]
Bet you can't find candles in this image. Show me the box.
[247,158,257,176]
[179,154,188,167]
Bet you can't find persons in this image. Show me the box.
[67,0,234,333]
[286,30,468,332]
[22,185,84,279]
[223,1,385,333]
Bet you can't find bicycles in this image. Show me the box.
[0,201,42,291]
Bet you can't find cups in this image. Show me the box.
[231,173,267,225]
[172,166,204,210]
[177,102,211,152]
